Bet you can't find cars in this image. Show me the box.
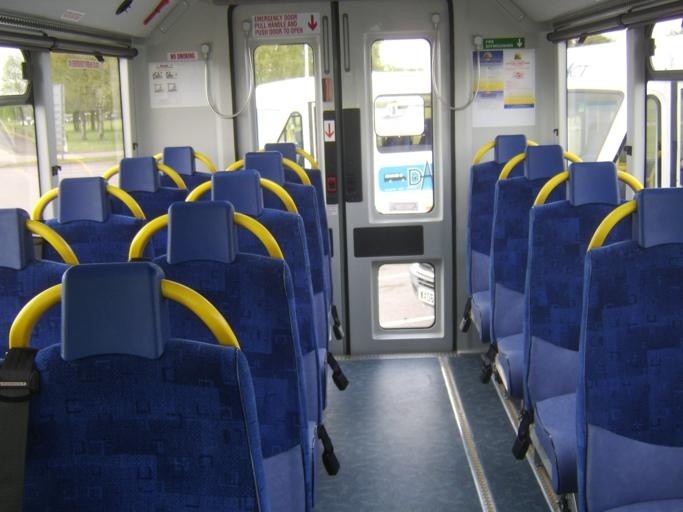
[408,262,438,308]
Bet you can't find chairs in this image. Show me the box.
[576,187,682,512]
[126,199,313,512]
[183,170,322,478]
[0,138,348,412]
[489,145,583,403]
[467,133,541,345]
[520,160,644,496]
[20,259,268,511]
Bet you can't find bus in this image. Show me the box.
[556,32,682,216]
[249,64,432,220]
